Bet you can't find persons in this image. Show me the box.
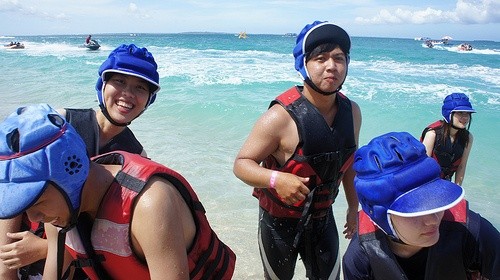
[0,44,161,280]
[0,103,236,280]
[335,131,500,280]
[420,93,476,186]
[86,35,92,44]
[233,21,362,280]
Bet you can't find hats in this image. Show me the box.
[293,20,351,80]
[354,131,465,235]
[0,103,89,222]
[95,44,161,110]
[442,92,477,124]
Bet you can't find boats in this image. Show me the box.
[416,37,472,51]
[4,44,25,49]
[85,40,101,50]
[239,32,246,39]
[282,33,298,38]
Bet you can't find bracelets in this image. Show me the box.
[270,171,277,187]
